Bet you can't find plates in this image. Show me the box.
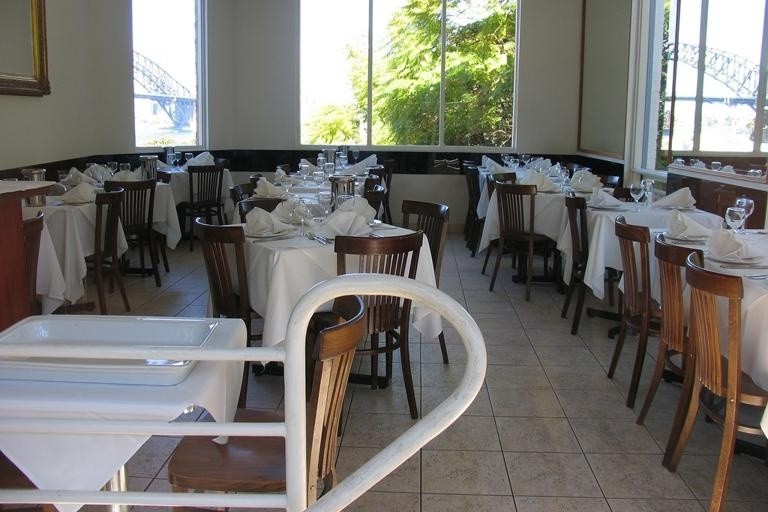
[253,194,281,199]
[664,233,705,242]
[586,204,623,209]
[62,201,91,205]
[368,219,381,226]
[244,231,288,238]
[664,204,696,211]
[565,187,591,193]
[0,313,224,386]
[704,251,768,265]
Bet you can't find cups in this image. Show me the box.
[746,169,762,178]
[58,174,72,192]
[86,162,131,181]
[20,169,47,207]
[139,155,158,183]
[675,157,722,171]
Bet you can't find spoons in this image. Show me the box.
[305,231,328,244]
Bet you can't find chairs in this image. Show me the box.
[176,166,224,253]
[168,152,229,166]
[608,214,687,409]
[145,170,172,273]
[229,163,293,224]
[560,196,624,336]
[104,179,162,293]
[662,251,764,512]
[402,199,450,366]
[306,230,424,438]
[489,183,563,300]
[167,295,367,512]
[463,160,516,275]
[195,216,265,409]
[635,233,716,427]
[362,158,397,226]
[85,187,132,315]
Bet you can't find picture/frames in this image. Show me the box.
[0,1,51,97]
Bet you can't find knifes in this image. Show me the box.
[718,264,768,270]
[253,236,290,243]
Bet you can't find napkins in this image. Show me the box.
[704,229,749,264]
[244,206,296,236]
[667,208,713,240]
[652,187,697,209]
[319,212,373,240]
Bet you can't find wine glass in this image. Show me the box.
[168,152,194,172]
[281,174,293,193]
[499,152,570,190]
[300,152,349,193]
[285,191,355,237]
[352,150,359,164]
[630,179,655,215]
[736,198,755,234]
[725,207,746,236]
